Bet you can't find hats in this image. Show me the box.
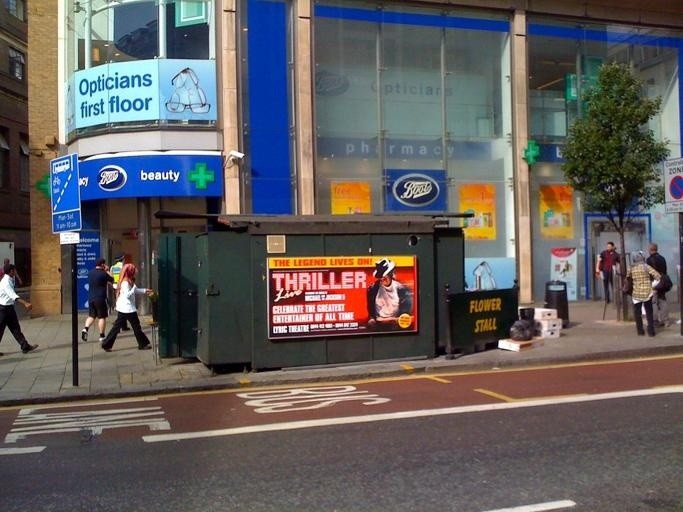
[113,251,126,261]
[374,258,397,278]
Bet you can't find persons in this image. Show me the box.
[646,244,667,327]
[0,257,23,287]
[621,249,662,336]
[100,262,153,353]
[109,253,130,333]
[365,257,413,331]
[0,263,38,355]
[81,257,114,343]
[593,241,621,305]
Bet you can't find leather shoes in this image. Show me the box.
[29,344,38,350]
[81,321,154,352]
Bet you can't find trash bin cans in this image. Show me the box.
[544,280,568,328]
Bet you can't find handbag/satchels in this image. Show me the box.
[653,273,672,292]
[622,277,633,296]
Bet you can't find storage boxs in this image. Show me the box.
[497,300,565,353]
